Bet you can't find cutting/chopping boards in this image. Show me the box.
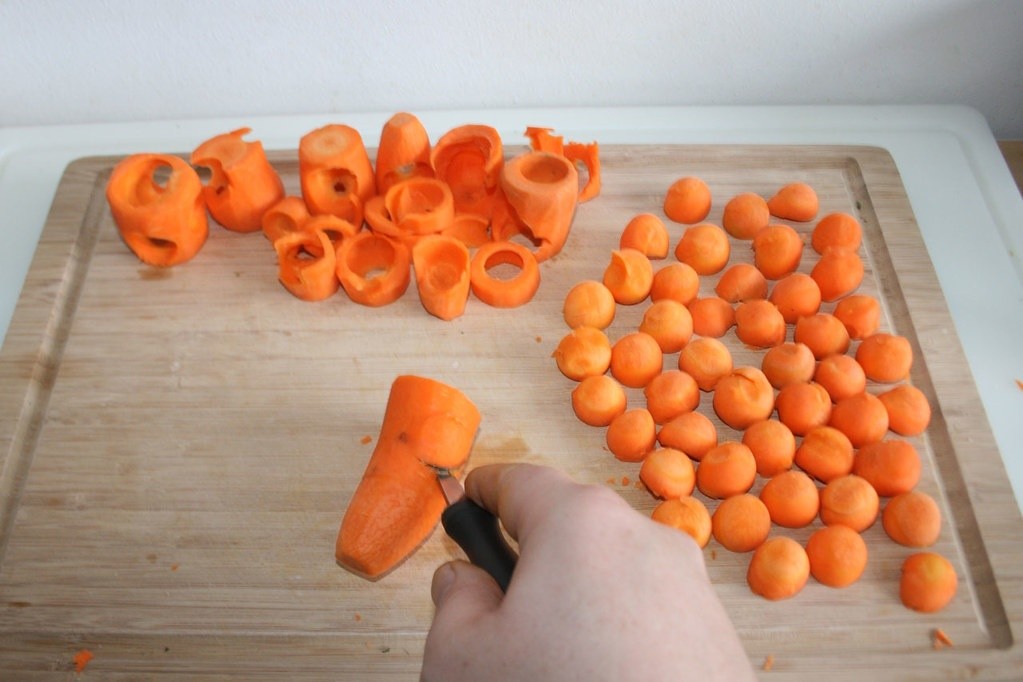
[0,146,1023,682]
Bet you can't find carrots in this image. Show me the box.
[105,112,601,318]
[334,374,481,578]
[556,178,962,615]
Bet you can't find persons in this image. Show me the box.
[418,462,758,682]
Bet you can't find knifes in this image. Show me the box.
[434,467,519,594]
